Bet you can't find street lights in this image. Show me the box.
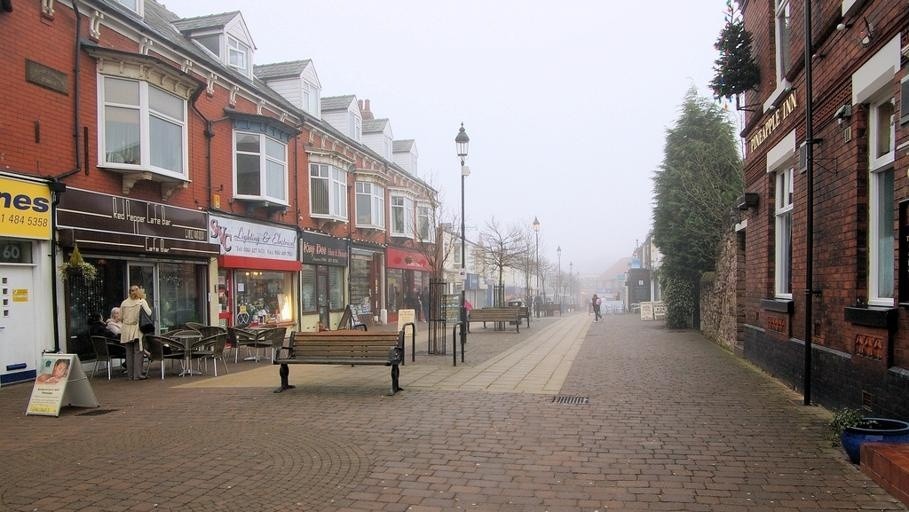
[454,123,472,364]
[554,245,562,317]
[531,216,539,318]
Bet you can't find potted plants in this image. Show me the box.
[825,405,909,464]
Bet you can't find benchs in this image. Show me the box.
[467,304,561,333]
[273,330,404,395]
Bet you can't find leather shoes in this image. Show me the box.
[132,375,149,381]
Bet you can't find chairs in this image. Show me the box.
[91,322,286,381]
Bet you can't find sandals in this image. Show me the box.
[139,307,155,334]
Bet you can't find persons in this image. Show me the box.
[35,358,70,385]
[591,294,603,322]
[106,302,123,336]
[89,309,154,352]
[119,283,152,380]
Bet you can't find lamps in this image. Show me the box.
[837,24,853,30]
[770,106,780,110]
[812,54,824,59]
[785,87,797,92]
